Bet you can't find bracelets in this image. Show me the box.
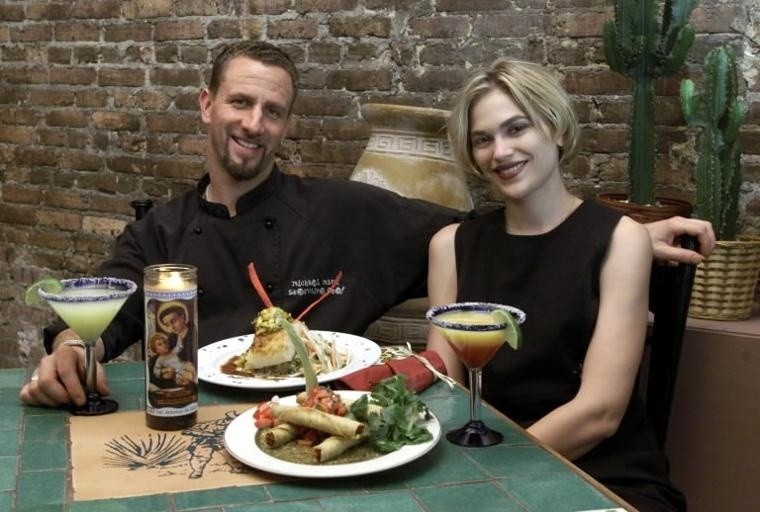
[51,339,88,353]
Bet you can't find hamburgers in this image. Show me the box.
[237,308,325,378]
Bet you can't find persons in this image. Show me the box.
[148,306,198,391]
[17,39,718,407]
[150,332,197,386]
[422,54,689,512]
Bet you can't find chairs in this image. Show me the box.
[610,232,689,512]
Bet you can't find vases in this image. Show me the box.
[346,100,475,352]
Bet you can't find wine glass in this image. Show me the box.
[38,277,141,417]
[425,303,529,449]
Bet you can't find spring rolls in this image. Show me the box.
[311,402,380,464]
[264,424,308,447]
[264,403,362,439]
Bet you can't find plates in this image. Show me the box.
[195,328,383,393]
[222,388,443,479]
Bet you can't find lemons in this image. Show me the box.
[489,309,524,352]
[23,278,63,306]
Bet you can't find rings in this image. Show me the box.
[28,374,39,384]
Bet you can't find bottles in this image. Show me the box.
[141,263,200,431]
